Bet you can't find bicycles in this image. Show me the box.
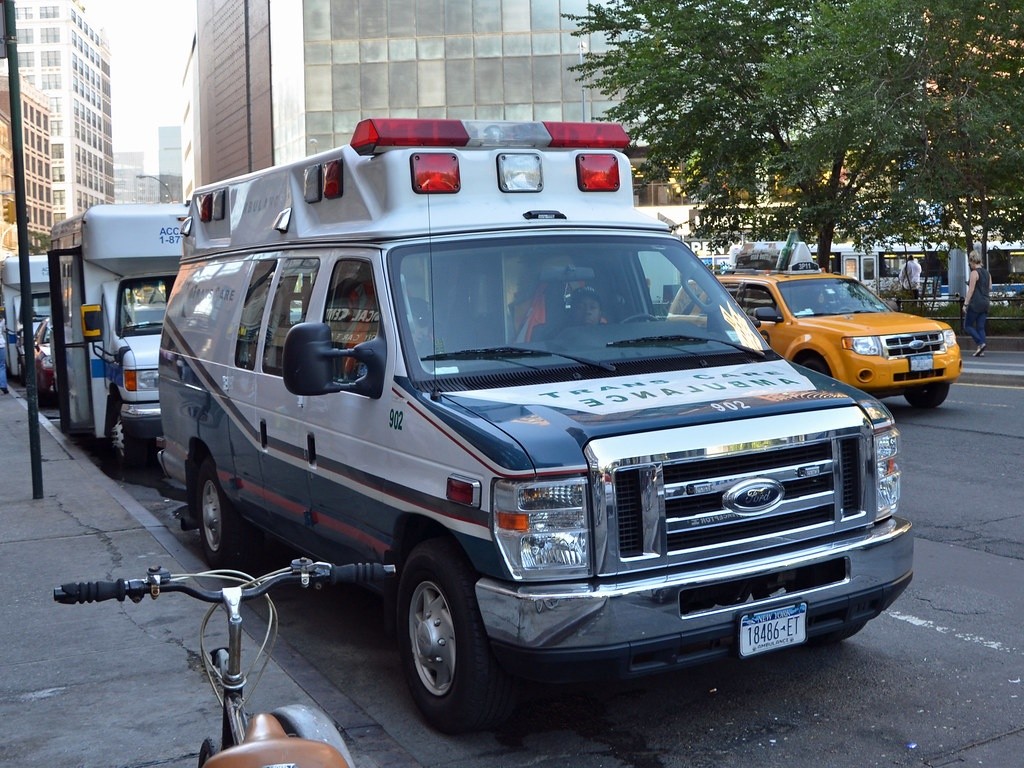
[51,554,396,768]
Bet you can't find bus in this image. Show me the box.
[731,237,1024,314]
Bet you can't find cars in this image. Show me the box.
[288,299,303,324]
[33,316,60,409]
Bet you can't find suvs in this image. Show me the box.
[661,270,962,411]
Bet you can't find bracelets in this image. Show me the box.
[964,304,969,306]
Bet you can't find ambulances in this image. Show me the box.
[156,118,917,740]
[46,202,223,468]
[0,254,74,387]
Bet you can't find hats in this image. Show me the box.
[570,285,602,307]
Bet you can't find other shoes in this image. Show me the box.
[972,343,986,356]
[1,388,8,394]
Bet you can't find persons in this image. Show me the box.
[569,287,604,327]
[962,250,992,357]
[899,254,921,307]
[0,312,9,394]
[409,297,438,346]
